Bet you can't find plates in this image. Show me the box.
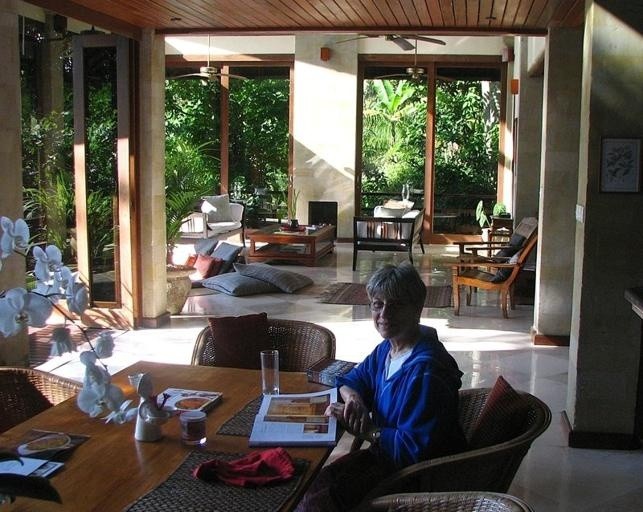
[16,433,70,457]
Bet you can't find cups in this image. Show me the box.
[178,410,208,446]
[260,350,280,399]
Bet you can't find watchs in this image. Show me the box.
[371,430,380,443]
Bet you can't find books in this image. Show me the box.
[154,386,224,417]
[306,356,359,389]
[249,388,339,446]
[0,428,90,491]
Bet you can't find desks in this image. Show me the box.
[485,214,513,231]
[485,229,512,271]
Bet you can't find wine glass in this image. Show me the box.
[401,183,409,209]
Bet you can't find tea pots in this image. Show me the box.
[289,219,299,228]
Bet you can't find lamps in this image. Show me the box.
[320,45,330,63]
[507,78,518,96]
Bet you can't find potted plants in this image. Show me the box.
[473,200,492,242]
[163,166,212,318]
[281,185,305,229]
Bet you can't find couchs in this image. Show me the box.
[349,207,427,272]
[174,193,245,249]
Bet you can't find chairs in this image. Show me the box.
[452,215,537,293]
[187,312,337,380]
[0,363,84,433]
[450,229,541,323]
[360,490,536,512]
[342,372,556,496]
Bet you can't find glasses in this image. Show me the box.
[369,301,409,312]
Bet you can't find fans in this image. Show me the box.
[338,31,448,53]
[372,37,455,90]
[172,33,254,90]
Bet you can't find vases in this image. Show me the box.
[132,399,166,440]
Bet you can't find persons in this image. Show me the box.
[301,265,463,511]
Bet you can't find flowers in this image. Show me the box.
[0,207,170,420]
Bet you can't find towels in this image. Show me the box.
[121,440,310,511]
[193,446,294,485]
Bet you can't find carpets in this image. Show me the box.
[319,278,466,310]
[26,322,128,370]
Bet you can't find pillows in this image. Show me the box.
[199,310,271,372]
[198,193,232,224]
[374,206,406,219]
[383,199,414,212]
[169,237,314,297]
[471,372,530,444]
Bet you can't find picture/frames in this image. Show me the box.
[598,132,641,197]
